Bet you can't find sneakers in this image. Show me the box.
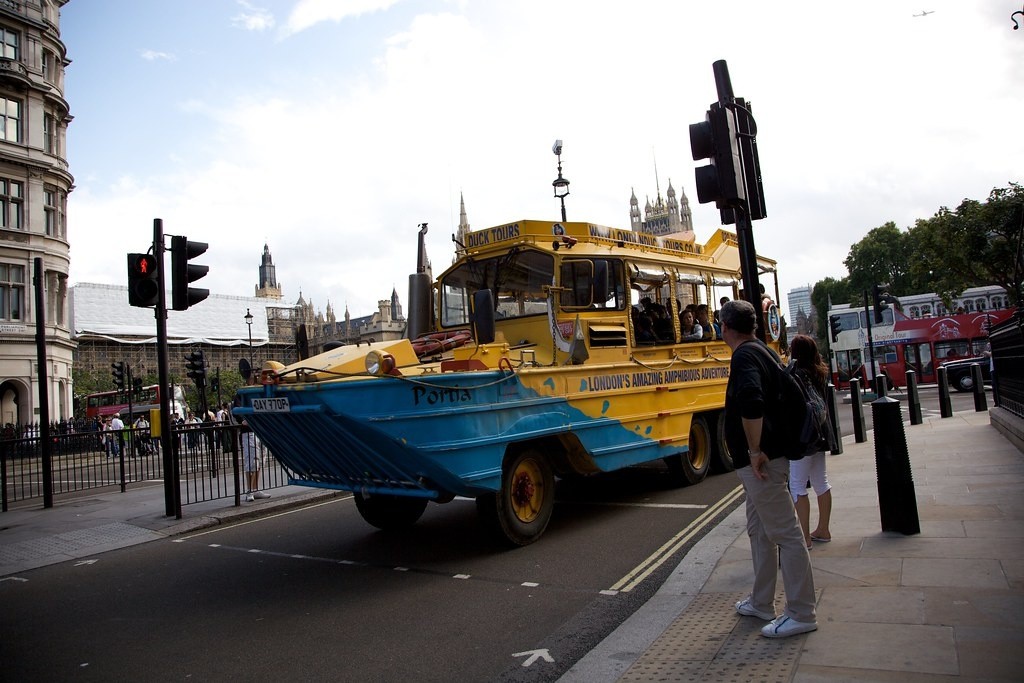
[734,598,777,621]
[254,491,271,498]
[762,613,817,637]
[246,493,255,502]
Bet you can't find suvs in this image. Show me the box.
[939,343,992,392]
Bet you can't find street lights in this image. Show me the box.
[552,139,571,221]
[244,308,254,369]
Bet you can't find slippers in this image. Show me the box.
[807,545,813,549]
[810,534,831,542]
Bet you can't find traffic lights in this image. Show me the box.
[184,349,206,380]
[128,253,160,307]
[690,102,746,206]
[211,377,219,392]
[830,315,842,342]
[111,362,125,390]
[132,377,143,395]
[873,282,889,324]
[172,235,210,311]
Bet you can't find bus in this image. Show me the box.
[86,382,188,424]
[827,281,1024,390]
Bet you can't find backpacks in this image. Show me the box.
[735,342,815,460]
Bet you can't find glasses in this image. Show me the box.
[789,347,792,352]
[141,417,144,418]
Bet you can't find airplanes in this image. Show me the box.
[912,9,935,17]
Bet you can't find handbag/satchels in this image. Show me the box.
[801,368,827,424]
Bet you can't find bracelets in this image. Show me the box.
[748,449,761,457]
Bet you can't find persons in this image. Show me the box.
[946,348,981,357]
[3,423,16,439]
[982,342,998,408]
[955,307,965,315]
[216,402,240,451]
[666,297,720,340]
[170,413,184,437]
[134,414,150,456]
[141,433,158,452]
[184,412,203,451]
[979,308,983,313]
[631,297,673,346]
[123,417,131,456]
[67,417,77,442]
[90,416,103,452]
[725,323,818,638]
[202,404,215,449]
[789,334,832,549]
[22,425,35,445]
[102,412,126,458]
[851,357,860,364]
[739,284,773,312]
[238,415,271,502]
